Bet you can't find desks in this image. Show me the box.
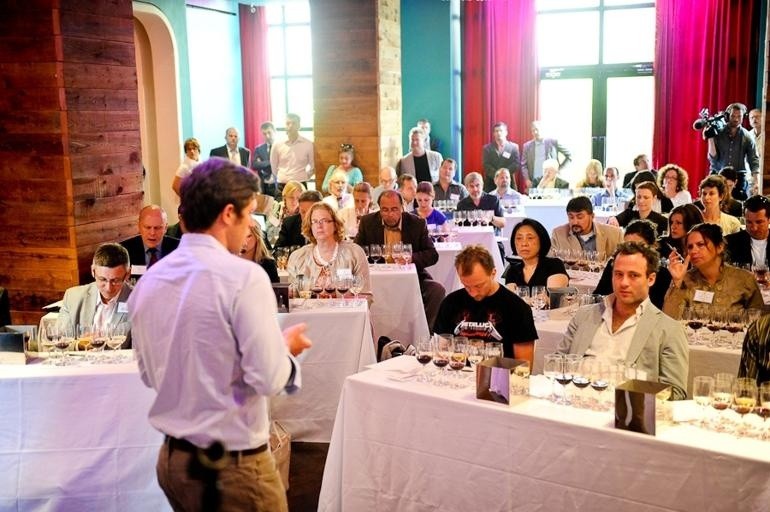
[0,197,770,512]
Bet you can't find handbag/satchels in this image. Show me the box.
[270,420,291,491]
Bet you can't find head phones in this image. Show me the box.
[724,103,749,122]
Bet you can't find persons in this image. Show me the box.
[554,241,689,401]
[553,154,770,322]
[253,122,278,195]
[738,307,769,400]
[239,120,568,359]
[56,244,136,349]
[210,128,252,167]
[120,203,182,269]
[430,245,539,374]
[694,102,762,199]
[270,113,315,190]
[171,136,204,197]
[124,154,315,512]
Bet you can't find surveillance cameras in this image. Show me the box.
[250,7,256,14]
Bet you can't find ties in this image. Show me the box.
[148,249,157,269]
[230,151,236,162]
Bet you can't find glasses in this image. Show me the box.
[311,219,333,225]
[93,271,126,286]
[340,144,354,149]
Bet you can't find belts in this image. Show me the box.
[165,435,268,459]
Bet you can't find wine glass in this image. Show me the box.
[734,379,757,439]
[447,210,495,229]
[548,247,770,298]
[512,284,762,350]
[428,196,521,212]
[361,242,414,271]
[715,373,732,424]
[693,377,714,426]
[555,359,571,405]
[274,245,301,268]
[40,321,127,367]
[594,361,607,411]
[526,184,626,211]
[572,357,590,408]
[759,383,770,440]
[711,380,732,433]
[544,355,563,402]
[294,275,363,309]
